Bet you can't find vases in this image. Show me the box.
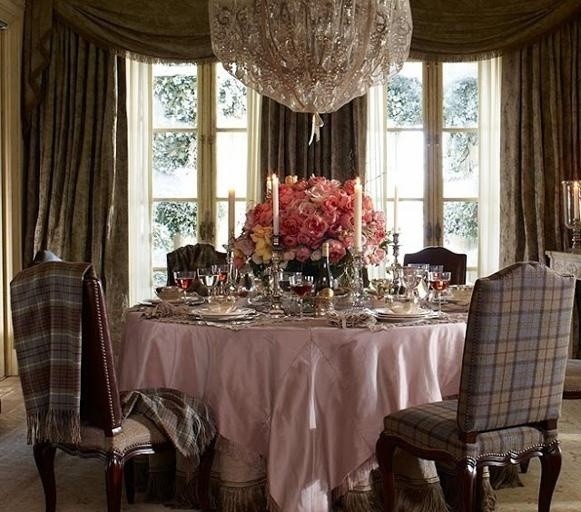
[249,250,353,297]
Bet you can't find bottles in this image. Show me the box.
[315,242,335,299]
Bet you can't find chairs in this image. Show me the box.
[166,244,228,296]
[372,260,576,512]
[403,246,466,285]
[9,262,218,512]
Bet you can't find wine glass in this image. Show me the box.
[561,179,581,255]
[173,261,452,323]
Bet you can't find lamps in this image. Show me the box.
[208,0,414,145]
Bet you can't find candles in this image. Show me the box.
[271,173,280,234]
[267,175,272,196]
[394,184,398,234]
[227,189,234,246]
[355,177,363,249]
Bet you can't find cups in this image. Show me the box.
[450,285,475,307]
[154,287,186,304]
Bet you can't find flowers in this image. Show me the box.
[230,173,393,279]
